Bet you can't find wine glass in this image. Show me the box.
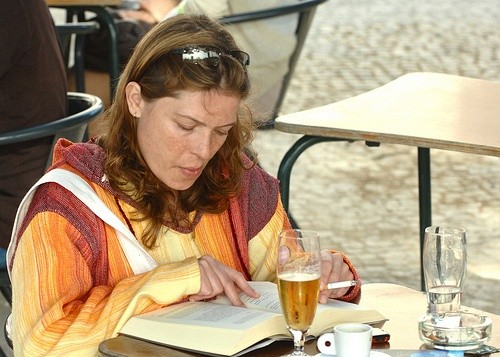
[278,229,321,357]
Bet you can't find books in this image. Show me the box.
[115,281,389,357]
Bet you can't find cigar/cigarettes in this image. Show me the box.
[324,280,358,290]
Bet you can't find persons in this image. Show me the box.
[68,0,301,150]
[6,12,361,357]
[0,0,66,271]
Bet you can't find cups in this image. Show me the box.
[422,224,466,312]
[333,323,374,357]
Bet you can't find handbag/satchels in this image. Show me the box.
[79,12,157,73]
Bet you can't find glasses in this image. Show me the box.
[168,44,251,69]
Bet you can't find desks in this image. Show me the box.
[99,283,500,357]
[274,70,499,293]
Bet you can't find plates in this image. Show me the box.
[313,349,392,357]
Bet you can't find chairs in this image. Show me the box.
[0,0,327,351]
